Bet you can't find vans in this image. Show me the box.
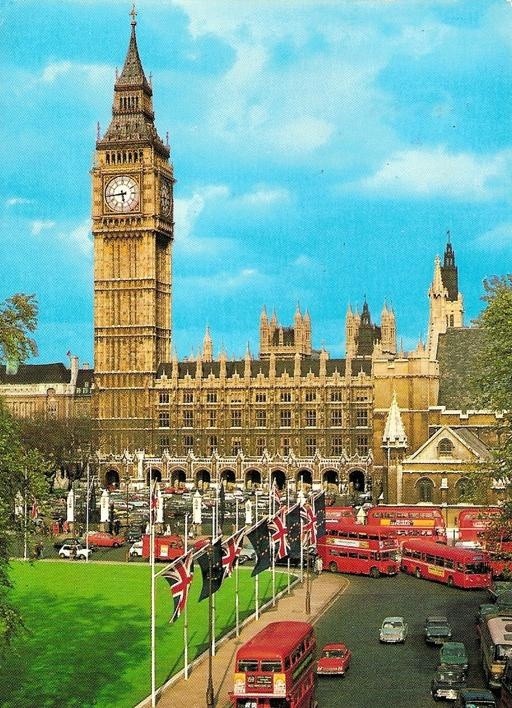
[454,541,484,553]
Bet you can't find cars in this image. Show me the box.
[420,581,512,708]
[316,643,354,677]
[377,615,410,644]
[9,473,384,568]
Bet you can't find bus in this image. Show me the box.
[226,621,320,708]
[313,507,511,591]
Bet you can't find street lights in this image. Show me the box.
[203,536,222,707]
[302,495,316,614]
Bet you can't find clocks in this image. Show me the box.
[160,181,172,218]
[103,174,140,213]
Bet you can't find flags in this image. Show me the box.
[160,551,194,622]
[299,507,318,548]
[312,492,328,540]
[271,483,282,505]
[194,538,225,604]
[218,529,246,581]
[266,508,292,563]
[246,518,274,579]
[31,499,38,520]
[284,504,302,561]
[151,483,158,527]
